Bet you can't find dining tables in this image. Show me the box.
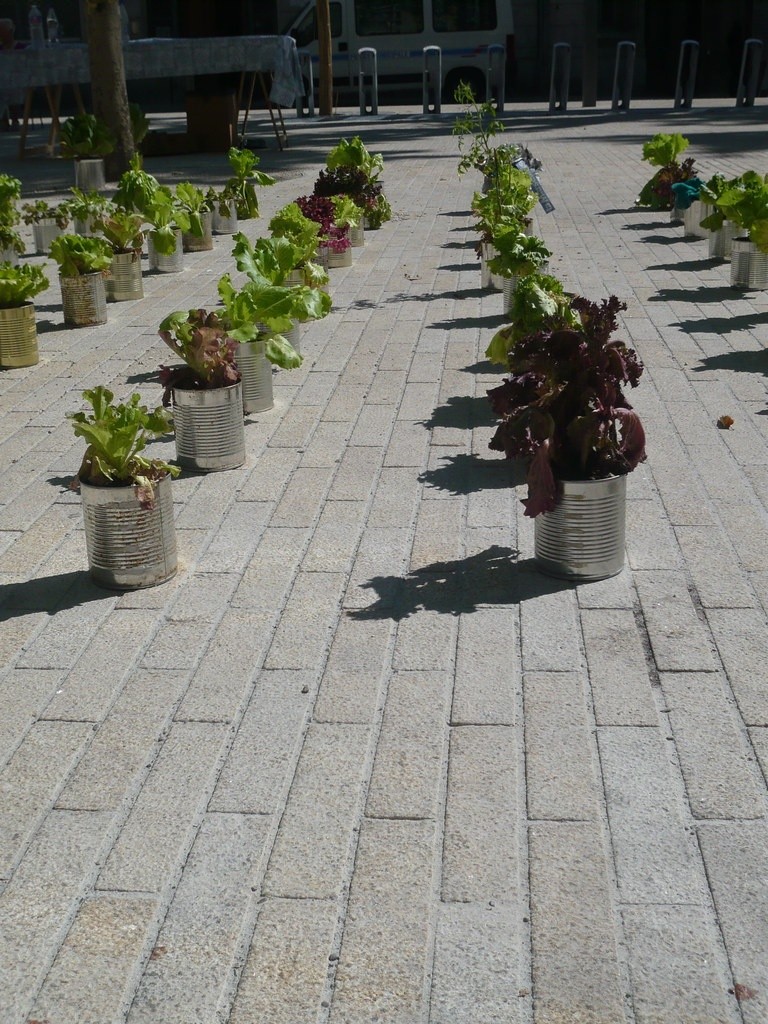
[0,35,303,161]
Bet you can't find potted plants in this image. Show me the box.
[484,223,554,318]
[49,233,114,327]
[176,179,215,253]
[0,261,50,369]
[62,383,185,590]
[153,309,258,479]
[93,214,157,302]
[268,203,329,318]
[294,191,365,267]
[671,178,712,239]
[218,232,332,360]
[651,158,699,221]
[469,191,526,293]
[63,187,109,238]
[716,170,768,291]
[111,152,162,217]
[698,172,749,258]
[495,171,539,236]
[326,136,392,230]
[137,192,191,274]
[204,184,241,235]
[20,201,73,256]
[59,114,117,190]
[213,271,304,413]
[313,165,367,247]
[485,295,654,581]
